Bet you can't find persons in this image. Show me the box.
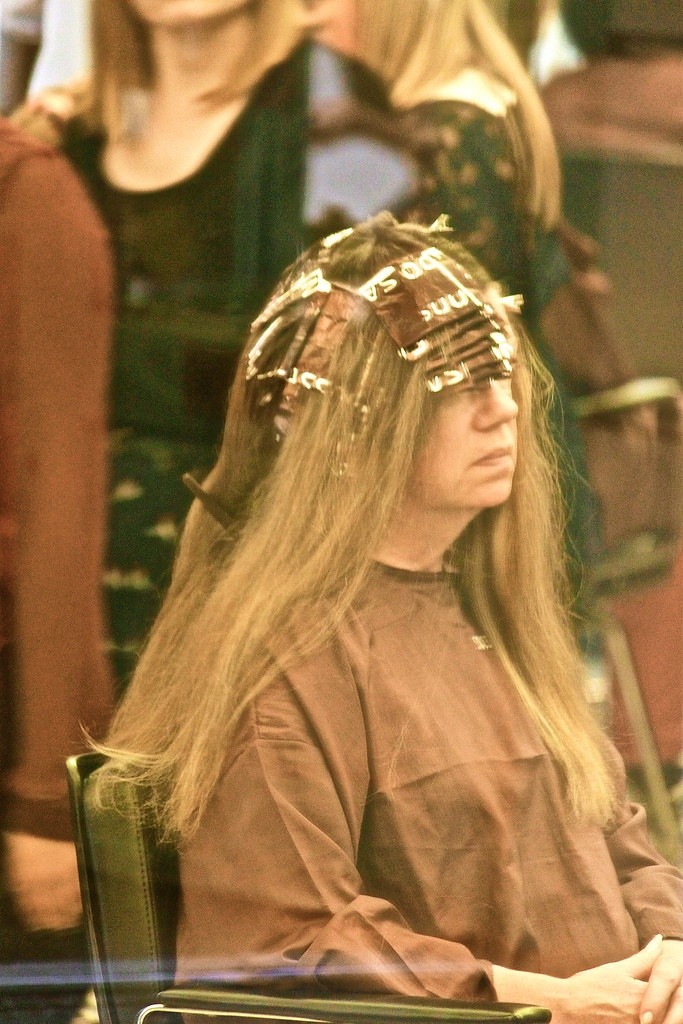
[84,210,683,1023]
[1,1,613,998]
[0,116,115,1024]
[305,0,608,655]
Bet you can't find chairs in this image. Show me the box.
[64,751,554,1024]
